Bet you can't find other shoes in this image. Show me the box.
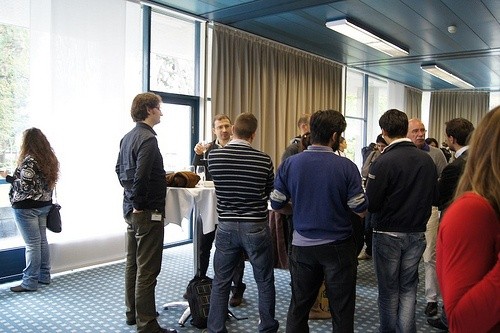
[358,253,372,259]
[230,293,242,306]
[11,286,28,291]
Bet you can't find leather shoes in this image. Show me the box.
[428,319,448,329]
[425,302,437,316]
[160,328,177,333]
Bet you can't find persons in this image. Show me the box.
[365,110,438,333]
[269,109,367,333]
[206,113,280,333]
[0,128,59,292]
[191,115,246,307]
[115,93,177,333]
[272,118,367,285]
[361,114,475,329]
[435,106,500,333]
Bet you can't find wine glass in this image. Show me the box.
[200,140,208,160]
[196,165,205,186]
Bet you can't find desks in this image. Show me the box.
[163,180,220,328]
[243,200,291,270]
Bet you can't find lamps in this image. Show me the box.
[325,19,410,57]
[420,65,475,90]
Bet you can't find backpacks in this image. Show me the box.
[186,275,230,329]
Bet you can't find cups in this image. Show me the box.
[187,166,195,173]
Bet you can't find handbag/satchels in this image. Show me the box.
[46,203,62,233]
[308,281,332,319]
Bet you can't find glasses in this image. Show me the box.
[154,106,161,110]
